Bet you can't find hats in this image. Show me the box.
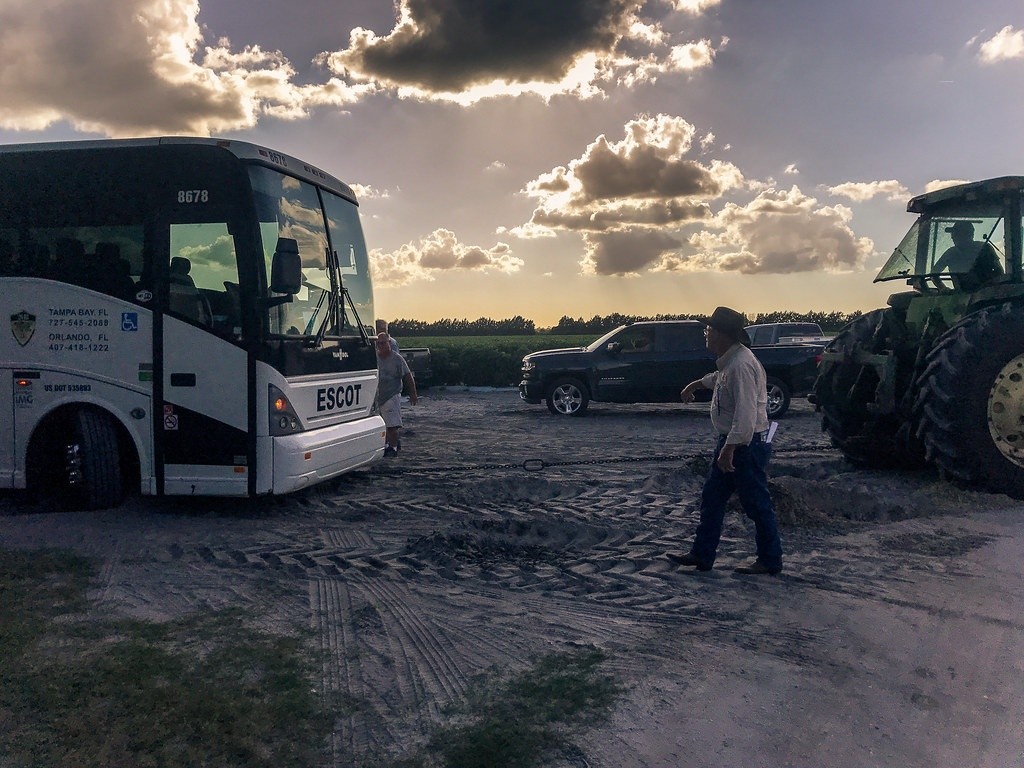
[694,306,752,346]
[944,220,975,232]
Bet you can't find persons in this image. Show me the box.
[376,331,418,457]
[931,221,1005,296]
[667,307,783,572]
[376,320,400,352]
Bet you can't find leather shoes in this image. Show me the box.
[665,552,715,571]
[733,560,784,575]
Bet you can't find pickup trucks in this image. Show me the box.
[518,319,837,419]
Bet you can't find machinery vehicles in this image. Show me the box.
[808,168,1023,497]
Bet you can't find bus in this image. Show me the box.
[0,135,389,512]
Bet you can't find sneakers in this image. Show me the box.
[384,446,399,459]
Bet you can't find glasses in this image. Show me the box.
[376,340,390,345]
[703,329,713,335]
